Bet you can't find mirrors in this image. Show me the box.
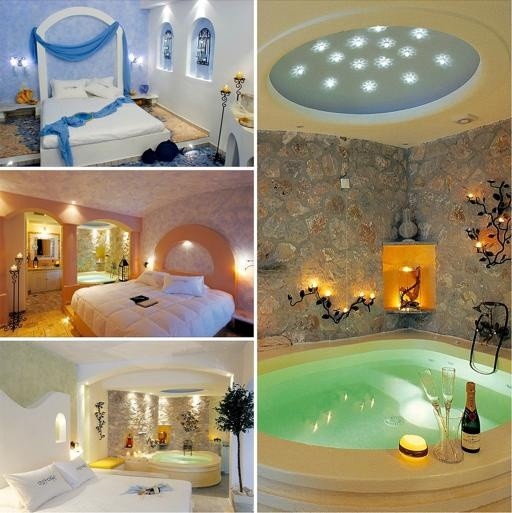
[27,231,59,267]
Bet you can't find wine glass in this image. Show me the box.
[417,367,464,464]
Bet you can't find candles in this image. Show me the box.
[15,251,22,257]
[236,71,242,80]
[223,85,229,93]
[10,264,16,270]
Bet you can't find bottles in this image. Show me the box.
[461,382,481,453]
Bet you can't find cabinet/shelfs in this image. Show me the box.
[28,269,60,293]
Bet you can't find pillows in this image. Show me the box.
[134,268,204,296]
[3,455,94,512]
[48,76,122,99]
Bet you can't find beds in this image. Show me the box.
[0,472,192,512]
[38,96,171,167]
[63,225,236,336]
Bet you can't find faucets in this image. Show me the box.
[478,321,492,338]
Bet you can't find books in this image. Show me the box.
[131,293,159,307]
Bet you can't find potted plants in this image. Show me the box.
[214,382,253,513]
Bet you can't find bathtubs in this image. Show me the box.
[76,270,119,284]
[124,449,222,489]
[257,326,512,511]
